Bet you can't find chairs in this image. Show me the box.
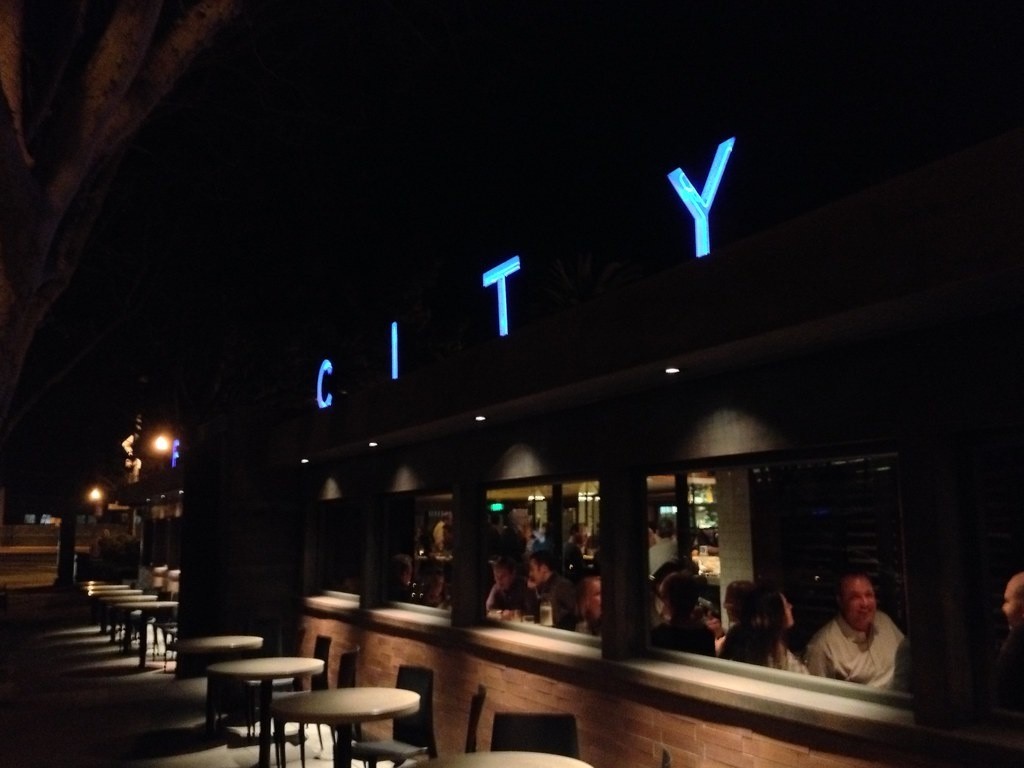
[116,580,672,768]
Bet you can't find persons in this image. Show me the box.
[648,519,808,674]
[385,513,456,608]
[486,511,602,637]
[989,571,1024,713]
[799,571,906,690]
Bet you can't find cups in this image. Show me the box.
[539,602,553,626]
[24,514,36,524]
[523,615,535,624]
[699,546,709,556]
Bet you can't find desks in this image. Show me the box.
[207,655,324,768]
[89,589,143,643]
[403,748,592,768]
[118,601,179,671]
[267,687,421,768]
[79,581,107,624]
[169,634,263,718]
[102,595,157,643]
[85,585,128,635]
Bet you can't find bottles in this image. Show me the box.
[488,606,521,624]
